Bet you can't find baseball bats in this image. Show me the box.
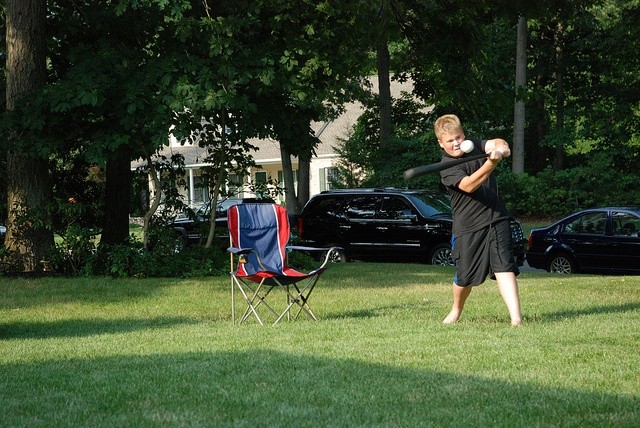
[403,154,490,181]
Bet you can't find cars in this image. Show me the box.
[526,207,640,275]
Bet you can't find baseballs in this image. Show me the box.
[460,140,475,153]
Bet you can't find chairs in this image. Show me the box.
[228,203,335,327]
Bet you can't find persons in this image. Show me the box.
[433,113,525,328]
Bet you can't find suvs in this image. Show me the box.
[297,188,524,266]
[163,198,276,254]
